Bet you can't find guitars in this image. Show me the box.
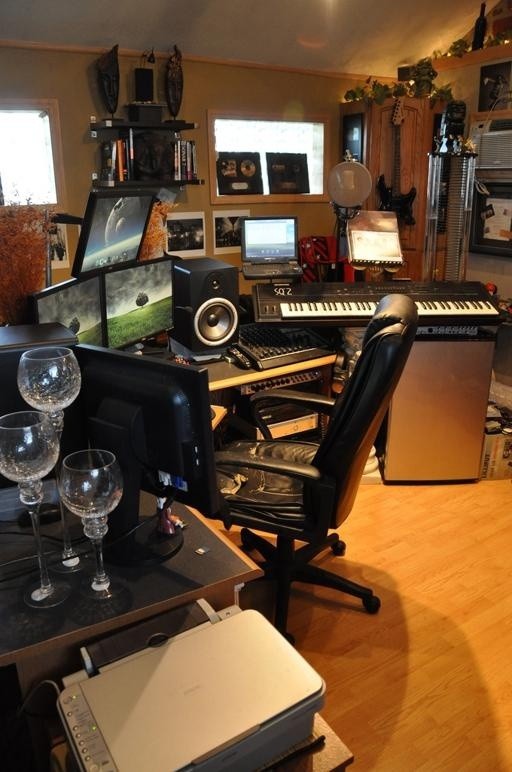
[375,99,418,226]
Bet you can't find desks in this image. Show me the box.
[1,474,264,711]
[44,606,356,770]
[170,327,336,447]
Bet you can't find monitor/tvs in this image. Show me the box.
[52,189,157,281]
[70,343,219,565]
[33,275,103,353]
[99,255,175,349]
[240,216,299,264]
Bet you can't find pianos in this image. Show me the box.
[251,280,504,328]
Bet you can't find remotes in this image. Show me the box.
[227,347,252,369]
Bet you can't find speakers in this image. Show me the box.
[0,321,78,416]
[174,258,240,357]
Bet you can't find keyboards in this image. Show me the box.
[242,262,303,280]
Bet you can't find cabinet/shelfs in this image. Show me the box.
[425,42,512,410]
[90,119,207,186]
[339,97,440,282]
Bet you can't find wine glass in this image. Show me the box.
[57,450,125,601]
[0,410,71,609]
[17,347,94,573]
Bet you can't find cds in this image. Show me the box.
[240,160,257,177]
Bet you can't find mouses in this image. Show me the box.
[18,503,63,528]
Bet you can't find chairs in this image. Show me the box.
[215,294,419,644]
